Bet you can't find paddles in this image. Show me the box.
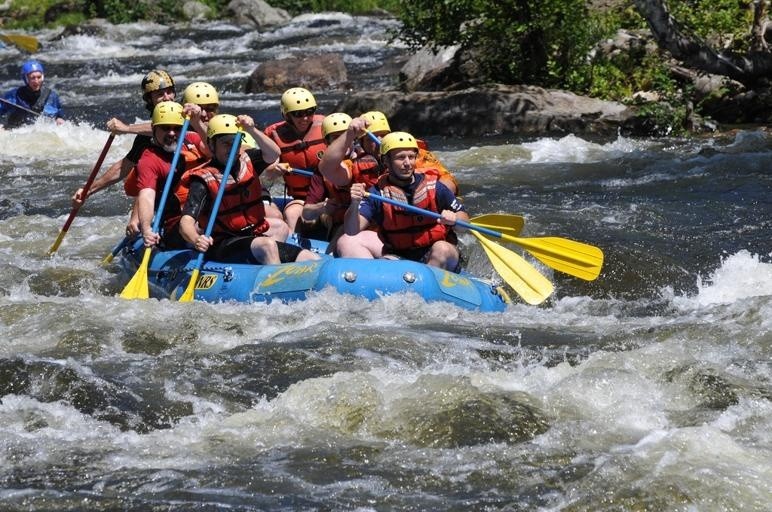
[362,191,604,306]
[120,109,192,302]
[181,125,242,302]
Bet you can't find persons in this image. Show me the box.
[71,66,469,277]
[1,59,65,130]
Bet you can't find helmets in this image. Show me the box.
[20,60,45,85]
[181,81,221,112]
[380,131,418,162]
[207,114,246,143]
[152,100,187,127]
[281,87,317,120]
[357,111,392,141]
[141,70,177,103]
[321,111,353,143]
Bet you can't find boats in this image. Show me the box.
[119,197,512,312]
